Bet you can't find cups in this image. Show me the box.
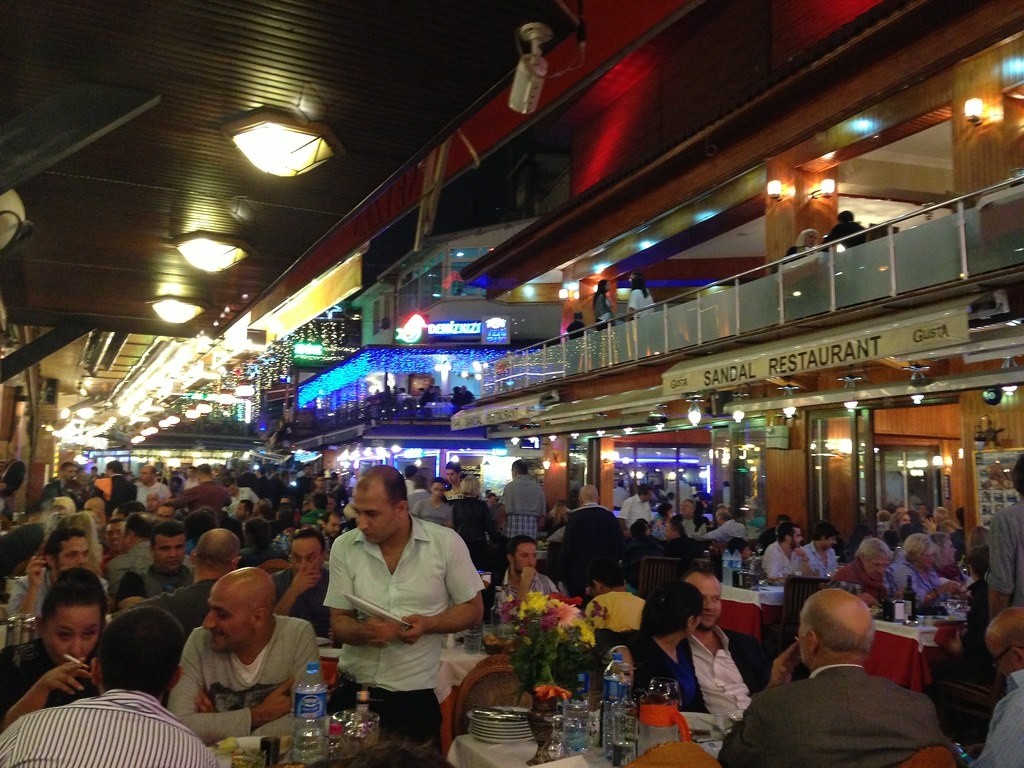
[561,702,590,753]
[947,599,966,613]
[328,710,380,760]
[649,678,682,710]
[211,747,266,768]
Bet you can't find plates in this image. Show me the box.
[340,591,411,627]
[678,709,744,735]
[767,581,784,586]
[916,615,967,624]
[215,737,290,755]
[467,706,535,745]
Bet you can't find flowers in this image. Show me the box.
[496,591,613,699]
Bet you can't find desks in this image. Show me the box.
[863,614,969,693]
[447,712,731,768]
[720,581,785,646]
[318,631,495,757]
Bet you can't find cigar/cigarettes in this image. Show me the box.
[794,637,800,640]
[63,654,89,669]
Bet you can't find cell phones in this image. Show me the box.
[954,743,975,766]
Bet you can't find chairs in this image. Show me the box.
[455,553,1007,768]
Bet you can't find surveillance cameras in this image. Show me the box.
[509,55,547,115]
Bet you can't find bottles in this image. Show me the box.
[749,551,759,574]
[326,725,349,768]
[571,652,639,767]
[903,575,916,621]
[722,549,742,586]
[289,662,329,765]
[356,691,370,712]
[463,585,508,656]
[5,613,38,646]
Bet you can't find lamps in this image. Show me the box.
[172,231,252,272]
[1001,385,1018,397]
[767,180,782,203]
[620,405,662,414]
[131,383,254,447]
[972,413,1007,451]
[687,361,930,426]
[964,97,982,127]
[145,297,211,324]
[549,414,595,425]
[543,452,560,470]
[220,110,344,178]
[0,188,35,255]
[812,178,836,200]
[559,289,570,302]
[79,330,130,375]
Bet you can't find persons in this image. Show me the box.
[787,229,819,263]
[821,211,866,255]
[0,453,1024,768]
[565,270,655,342]
[367,383,476,417]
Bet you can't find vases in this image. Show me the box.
[525,697,562,766]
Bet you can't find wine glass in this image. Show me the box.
[541,716,569,763]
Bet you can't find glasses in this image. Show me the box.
[924,552,937,558]
[868,559,889,569]
[991,646,1024,663]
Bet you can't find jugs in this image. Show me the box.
[637,704,690,757]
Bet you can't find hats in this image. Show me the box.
[656,501,669,513]
